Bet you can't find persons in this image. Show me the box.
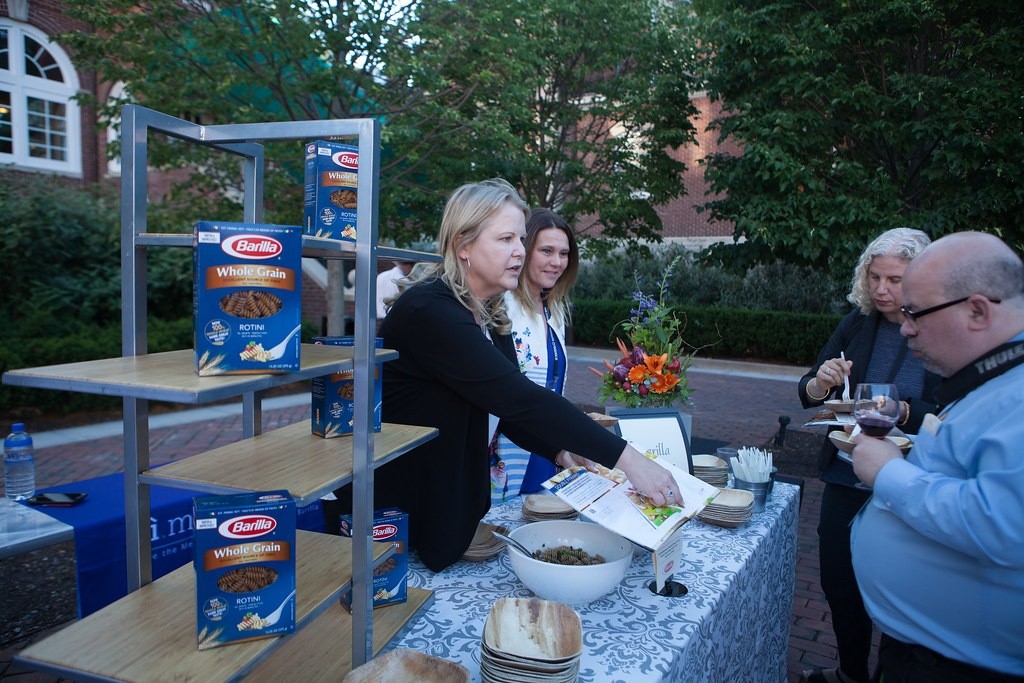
[848,230,1024,683]
[489,208,580,506]
[796,226,932,683]
[376,259,421,319]
[319,177,686,573]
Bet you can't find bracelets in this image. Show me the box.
[805,377,829,400]
[555,456,561,466]
[898,400,909,425]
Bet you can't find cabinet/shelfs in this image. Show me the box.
[4,105,442,683]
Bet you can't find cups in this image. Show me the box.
[733,474,772,514]
[766,466,777,501]
[717,448,738,473]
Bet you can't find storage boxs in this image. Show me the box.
[340,509,408,617]
[302,140,359,242]
[191,220,300,373]
[313,336,383,436]
[189,491,296,647]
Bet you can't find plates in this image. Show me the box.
[522,494,578,522]
[342,649,470,683]
[462,522,511,560]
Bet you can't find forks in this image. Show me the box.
[841,351,850,403]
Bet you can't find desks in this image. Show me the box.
[16,459,325,622]
[365,473,800,683]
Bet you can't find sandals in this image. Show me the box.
[800,667,870,683]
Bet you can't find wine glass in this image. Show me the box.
[853,383,899,490]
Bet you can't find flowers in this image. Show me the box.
[587,257,724,410]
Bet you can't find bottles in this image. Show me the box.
[3,423,35,501]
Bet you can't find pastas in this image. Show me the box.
[532,546,607,565]
[219,290,280,318]
[330,190,356,208]
[374,558,396,575]
[337,382,353,399]
[218,566,277,592]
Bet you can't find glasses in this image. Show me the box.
[900,296,1001,333]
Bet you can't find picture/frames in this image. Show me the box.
[609,408,694,477]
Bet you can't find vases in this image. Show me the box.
[655,405,692,453]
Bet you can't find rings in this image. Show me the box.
[663,490,673,497]
[825,367,831,374]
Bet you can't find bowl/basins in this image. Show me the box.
[692,454,728,485]
[824,399,853,412]
[828,430,913,455]
[506,519,635,606]
[698,488,755,528]
[481,597,583,683]
